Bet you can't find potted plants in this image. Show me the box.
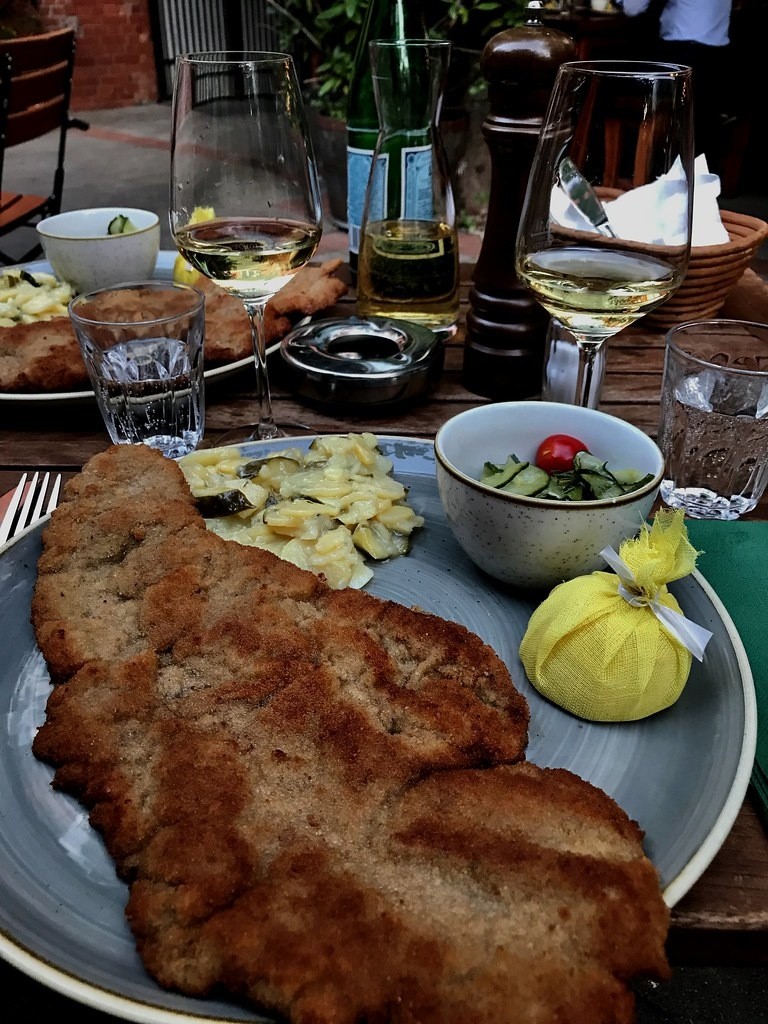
[258,0,530,234]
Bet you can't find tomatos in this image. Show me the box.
[535,434,589,474]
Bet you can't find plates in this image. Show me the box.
[0,251,313,400]
[0,433,757,1024]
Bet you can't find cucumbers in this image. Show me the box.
[479,453,657,501]
[106,214,139,235]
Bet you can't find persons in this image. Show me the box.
[623,0,733,204]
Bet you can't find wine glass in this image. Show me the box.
[170,52,325,443]
[514,59,692,410]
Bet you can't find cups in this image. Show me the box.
[657,319,768,521]
[68,279,206,460]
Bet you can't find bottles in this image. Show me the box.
[358,39,461,329]
[346,0,447,275]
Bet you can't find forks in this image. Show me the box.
[0,471,61,546]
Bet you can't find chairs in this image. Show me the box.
[0,28,75,268]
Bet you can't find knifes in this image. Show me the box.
[559,157,615,239]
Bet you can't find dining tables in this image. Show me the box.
[0,227,767,1024]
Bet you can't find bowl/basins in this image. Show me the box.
[36,207,160,293]
[434,400,664,583]
